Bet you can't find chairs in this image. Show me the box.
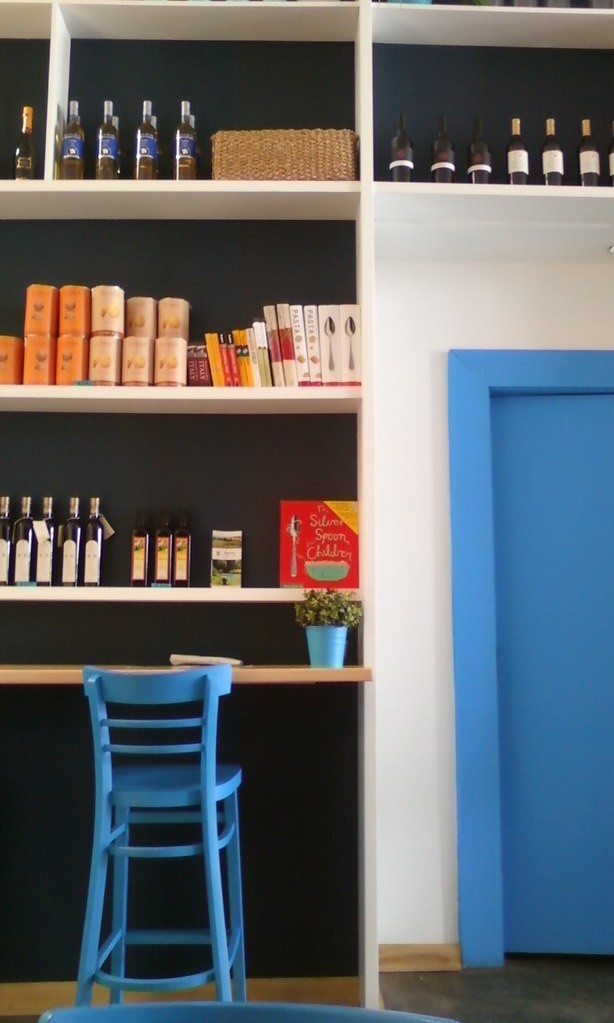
[80,664,249,1008]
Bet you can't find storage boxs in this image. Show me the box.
[279,499,359,591]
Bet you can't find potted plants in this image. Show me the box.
[295,587,361,669]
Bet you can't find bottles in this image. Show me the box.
[13,106,37,180]
[466,115,493,184]
[608,120,614,187]
[387,112,414,183]
[506,117,530,184]
[577,119,601,186]
[58,98,202,180]
[540,118,565,185]
[129,507,192,588]
[430,114,456,182]
[0,495,105,588]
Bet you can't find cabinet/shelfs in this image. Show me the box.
[0,1,614,990]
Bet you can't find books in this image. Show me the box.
[185,304,360,387]
